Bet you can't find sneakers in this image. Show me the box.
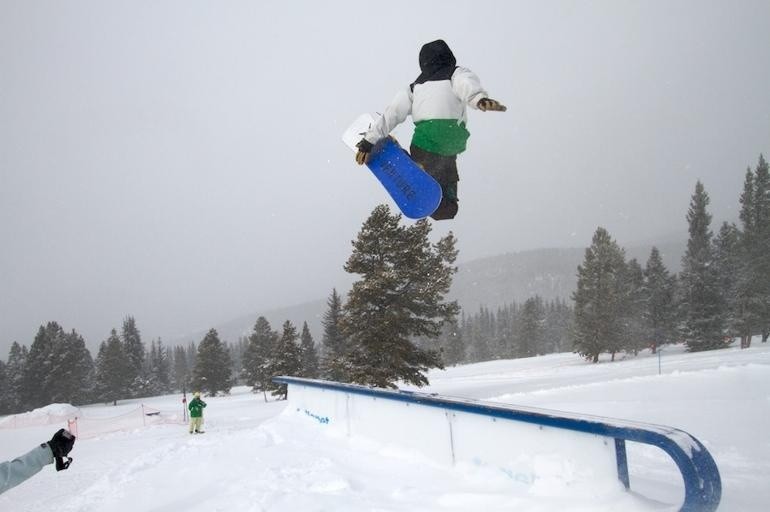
[387,133,399,145]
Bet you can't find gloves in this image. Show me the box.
[355,138,374,165]
[477,98,507,112]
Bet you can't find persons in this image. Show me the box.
[189,391,207,435]
[354,40,508,224]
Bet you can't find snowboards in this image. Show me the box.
[342,115,443,219]
